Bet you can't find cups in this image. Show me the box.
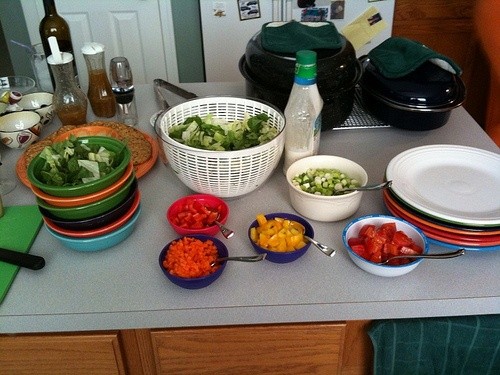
[116,95,137,127]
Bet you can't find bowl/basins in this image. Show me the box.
[53,126,119,143]
[0,75,36,95]
[15,92,58,126]
[0,111,42,150]
[247,212,315,264]
[286,154,368,223]
[341,214,430,277]
[27,136,143,253]
[167,194,230,237]
[158,235,229,290]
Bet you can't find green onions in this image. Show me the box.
[291,167,360,197]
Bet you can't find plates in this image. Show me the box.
[383,144,500,253]
[16,120,158,189]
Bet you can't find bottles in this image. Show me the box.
[283,50,324,177]
[80,44,117,118]
[47,52,88,126]
[38,0,81,91]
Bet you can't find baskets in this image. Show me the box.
[150,96,287,199]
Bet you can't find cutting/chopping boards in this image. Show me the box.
[0,205,43,303]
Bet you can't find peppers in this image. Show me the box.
[173,201,224,230]
[251,214,306,252]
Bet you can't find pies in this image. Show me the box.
[24,121,151,168]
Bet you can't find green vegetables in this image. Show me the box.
[169,113,278,151]
[38,134,128,187]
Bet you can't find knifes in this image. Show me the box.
[0,248,46,271]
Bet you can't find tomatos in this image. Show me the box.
[348,222,421,267]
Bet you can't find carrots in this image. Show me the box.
[162,235,221,279]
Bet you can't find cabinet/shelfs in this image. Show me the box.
[0,314,126,375]
[137,321,350,375]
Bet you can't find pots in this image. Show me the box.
[355,56,469,131]
[238,30,364,132]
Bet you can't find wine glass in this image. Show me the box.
[108,56,136,113]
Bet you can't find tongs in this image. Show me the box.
[153,78,198,111]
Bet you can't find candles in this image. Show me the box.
[47,36,60,61]
